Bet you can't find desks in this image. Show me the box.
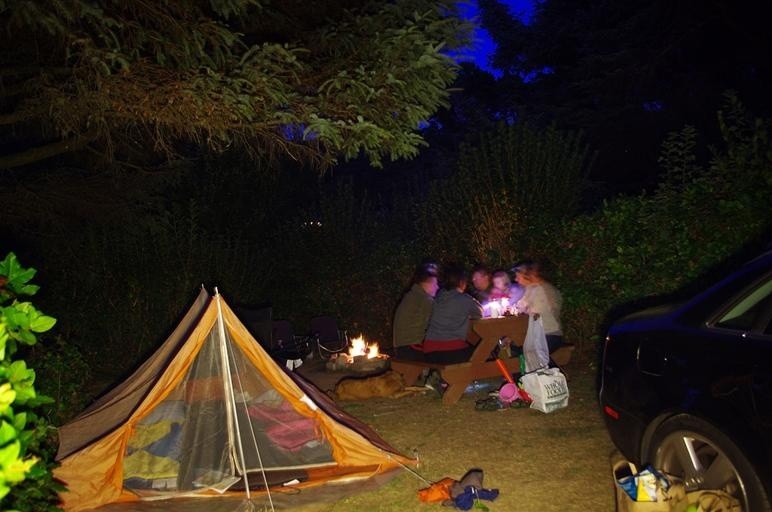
[390,314,572,407]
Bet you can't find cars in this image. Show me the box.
[598,249,772,512]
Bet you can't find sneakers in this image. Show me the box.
[423,377,444,399]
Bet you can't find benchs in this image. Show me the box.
[550,344,575,366]
[388,354,473,385]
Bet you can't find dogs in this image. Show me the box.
[327,368,430,401]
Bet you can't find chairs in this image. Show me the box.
[270,316,348,372]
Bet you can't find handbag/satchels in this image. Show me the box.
[522,368,570,413]
[613,461,741,511]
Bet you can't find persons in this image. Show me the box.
[470,269,493,303]
[422,271,501,364]
[391,274,439,360]
[392,257,444,329]
[487,270,524,303]
[511,258,568,381]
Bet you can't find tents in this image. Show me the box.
[53,283,423,511]
